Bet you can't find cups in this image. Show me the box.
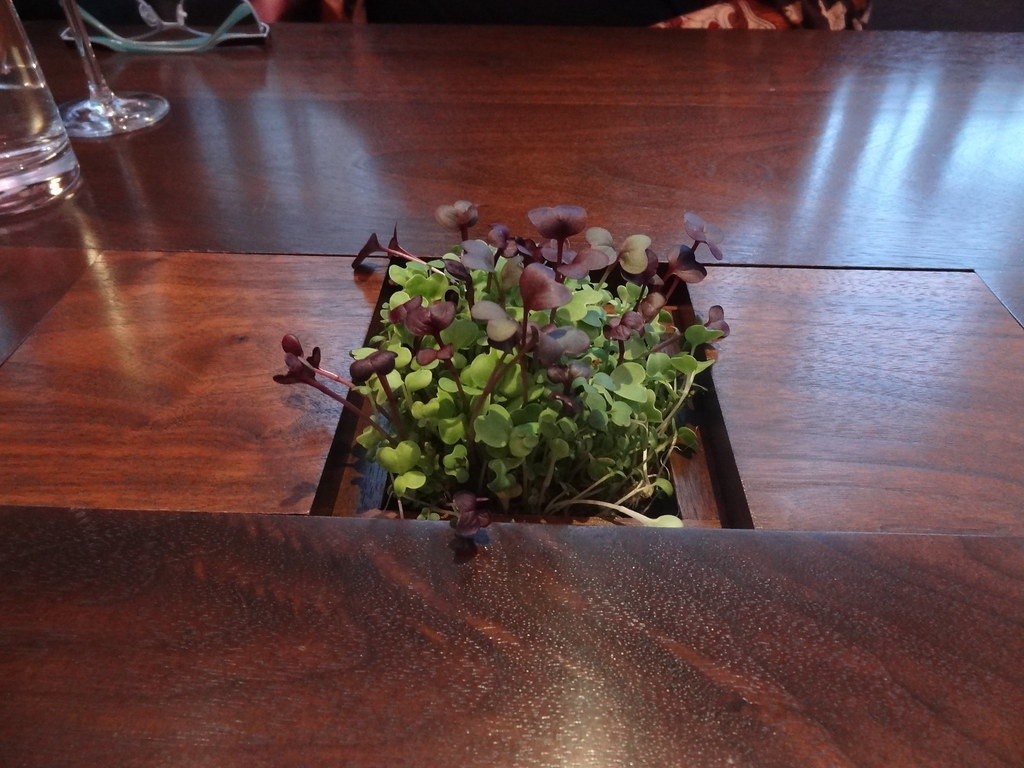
[0,0,80,216]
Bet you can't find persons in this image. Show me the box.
[251,0,879,31]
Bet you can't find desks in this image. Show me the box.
[1,28,1024,768]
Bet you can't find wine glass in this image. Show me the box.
[56,0,170,138]
